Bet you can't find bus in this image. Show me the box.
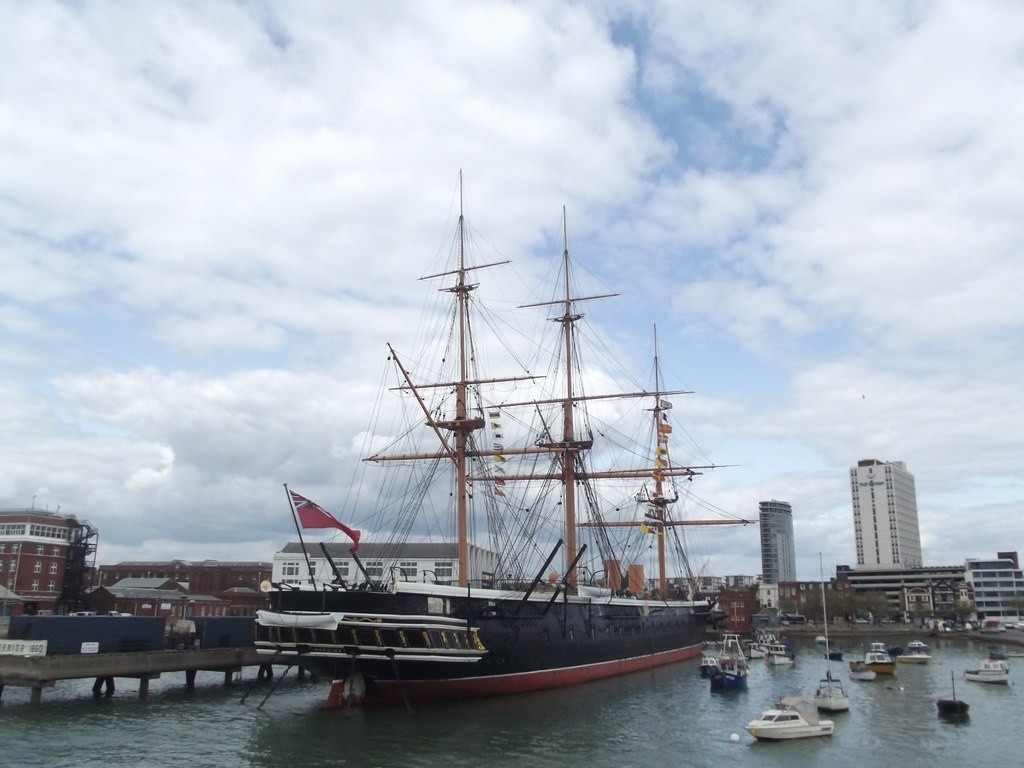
[782,615,807,624]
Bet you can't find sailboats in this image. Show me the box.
[250,164,761,700]
[814,550,849,713]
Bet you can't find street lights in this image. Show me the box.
[261,580,271,609]
[180,595,196,620]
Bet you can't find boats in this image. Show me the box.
[825,647,843,660]
[848,662,876,681]
[814,636,832,644]
[865,641,895,675]
[907,641,930,652]
[716,634,750,690]
[965,651,1010,683]
[700,656,717,677]
[897,650,932,664]
[742,631,794,665]
[937,669,970,718]
[887,644,904,656]
[743,697,835,740]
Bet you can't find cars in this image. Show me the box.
[944,620,1024,633]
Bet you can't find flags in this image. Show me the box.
[657,434,668,443]
[290,490,360,552]
[489,411,501,420]
[653,472,664,480]
[657,446,667,455]
[660,412,668,422]
[495,455,505,462]
[491,422,501,429]
[495,434,503,439]
[493,443,503,450]
[656,459,667,468]
[658,424,672,433]
